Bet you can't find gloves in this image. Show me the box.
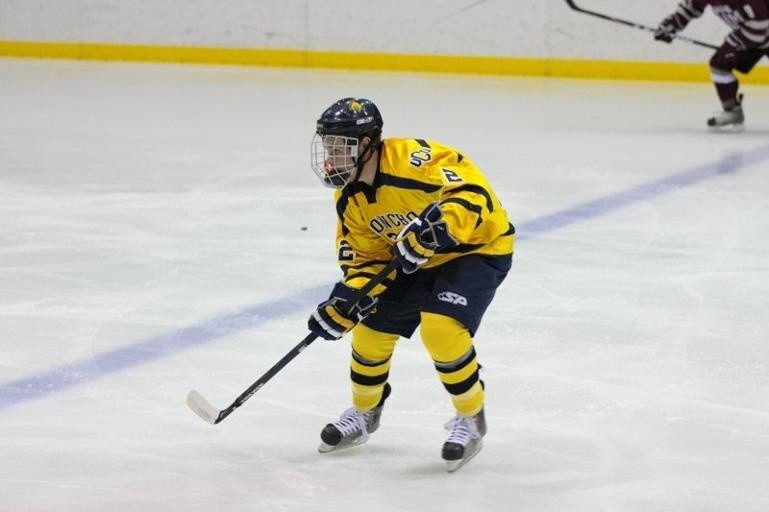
[393,202,460,274]
[655,15,685,43]
[715,41,739,65]
[307,282,380,341]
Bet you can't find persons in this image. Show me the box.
[301,97,517,463]
[653,1,769,127]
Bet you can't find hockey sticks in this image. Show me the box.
[185,253,400,426]
[565,0,724,51]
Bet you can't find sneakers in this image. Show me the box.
[707,103,744,126]
[320,383,391,445]
[441,406,488,460]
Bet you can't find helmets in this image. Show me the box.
[317,97,383,138]
